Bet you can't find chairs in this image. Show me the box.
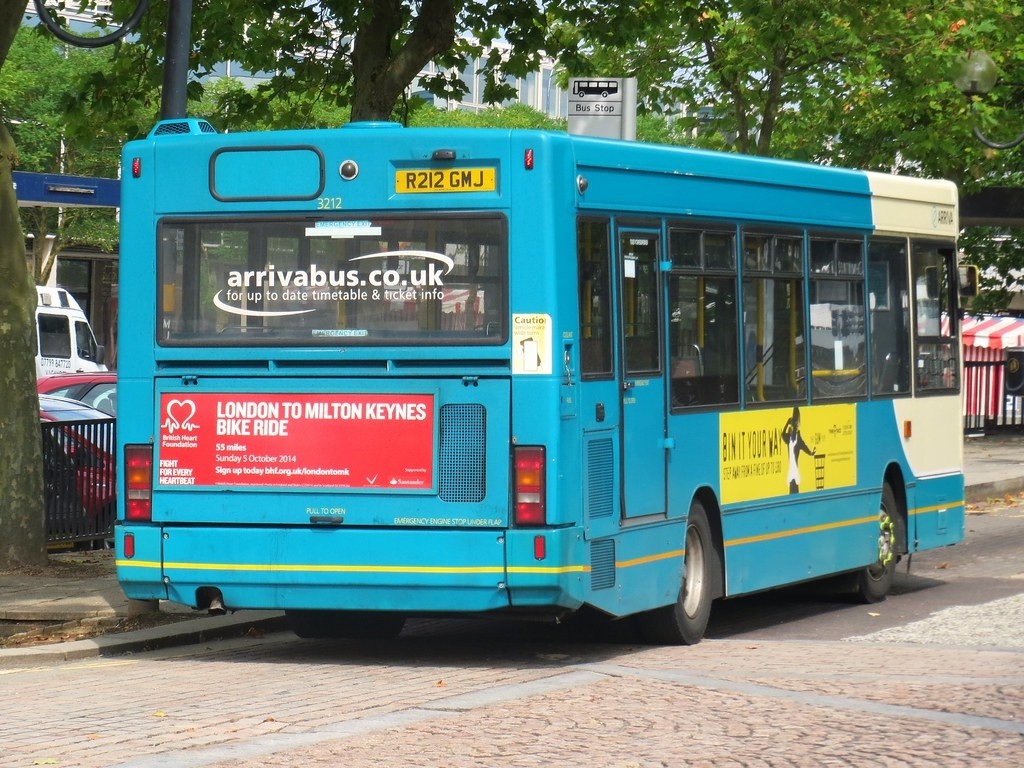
[97,399,115,417]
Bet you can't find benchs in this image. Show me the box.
[217,321,909,408]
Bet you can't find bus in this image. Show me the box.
[111,117,976,646]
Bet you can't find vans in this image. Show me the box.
[35,285,110,377]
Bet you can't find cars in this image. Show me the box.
[37,373,117,417]
[38,395,117,549]
